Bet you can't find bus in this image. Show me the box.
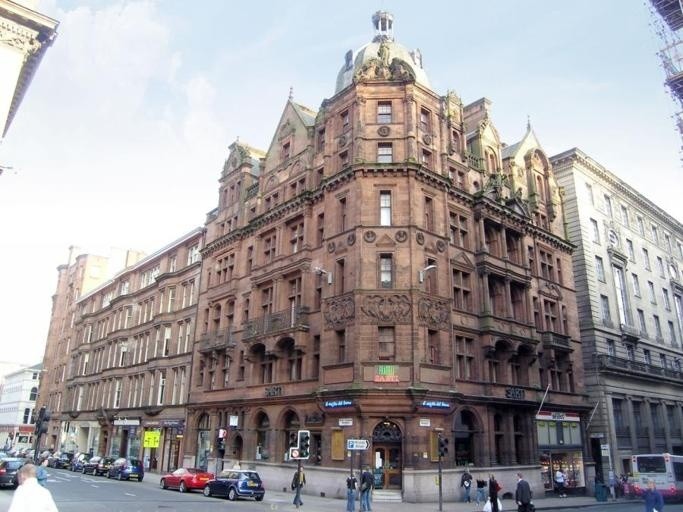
[631,453,682,503]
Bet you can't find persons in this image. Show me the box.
[36,459,50,486]
[360,465,374,512]
[292,466,305,506]
[488,473,498,512]
[347,473,358,512]
[515,470,532,512]
[555,470,567,499]
[7,464,58,511]
[461,468,473,503]
[645,478,665,512]
[475,475,486,509]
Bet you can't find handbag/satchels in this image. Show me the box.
[564,481,569,487]
[361,482,367,492]
[291,480,297,490]
[529,503,536,512]
[496,482,501,492]
[464,481,470,487]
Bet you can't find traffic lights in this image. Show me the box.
[300,434,309,457]
[438,437,448,457]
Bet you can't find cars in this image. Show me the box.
[0,447,143,489]
[160,467,214,493]
[203,469,264,501]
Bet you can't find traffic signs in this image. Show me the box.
[346,439,369,450]
